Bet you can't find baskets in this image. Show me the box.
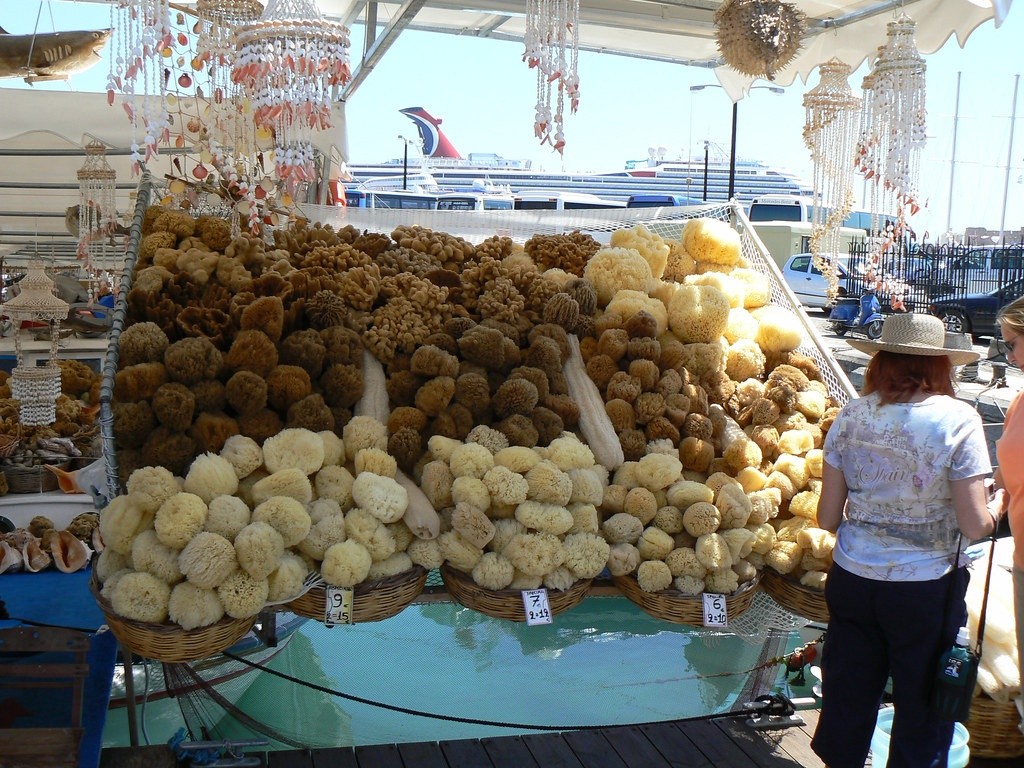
[611,570,760,627]
[67,426,101,450]
[962,695,1024,758]
[439,560,594,622]
[0,401,20,458]
[90,553,259,664]
[0,461,70,493]
[281,564,429,623]
[761,565,830,623]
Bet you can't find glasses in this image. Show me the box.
[997,333,1022,353]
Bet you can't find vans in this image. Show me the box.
[926,245,1024,297]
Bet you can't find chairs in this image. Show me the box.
[0,626,118,768]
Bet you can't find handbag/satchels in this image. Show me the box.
[923,640,979,723]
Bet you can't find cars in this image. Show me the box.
[929,277,1024,337]
[781,254,931,316]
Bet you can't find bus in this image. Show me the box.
[346,188,701,209]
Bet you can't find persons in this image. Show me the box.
[74,279,115,325]
[992,298,1024,737]
[810,314,1010,768]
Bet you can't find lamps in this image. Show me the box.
[801,25,862,309]
[522,0,578,153]
[76,139,120,307]
[231,0,351,162]
[194,0,265,240]
[3,227,70,428]
[106,0,174,183]
[855,0,926,314]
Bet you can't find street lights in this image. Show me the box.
[689,83,785,205]
[396,134,413,189]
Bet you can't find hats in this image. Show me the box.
[846,313,980,366]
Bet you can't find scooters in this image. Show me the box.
[828,280,885,340]
